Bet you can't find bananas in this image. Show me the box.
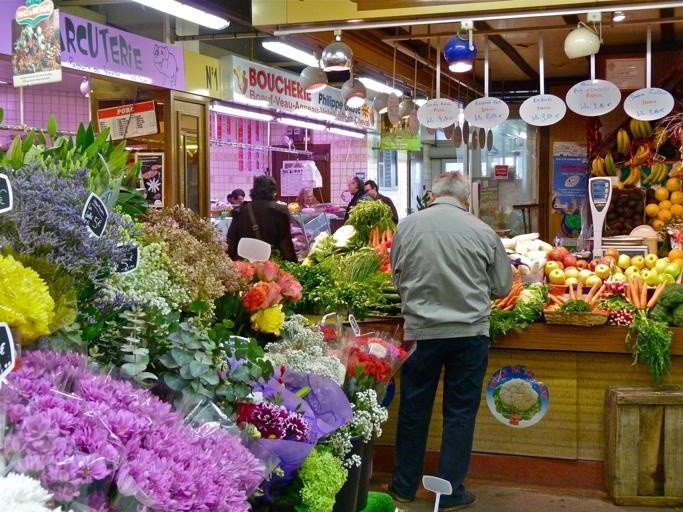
[592,116,669,191]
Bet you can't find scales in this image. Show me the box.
[588,177,648,260]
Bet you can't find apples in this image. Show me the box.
[545,247,683,288]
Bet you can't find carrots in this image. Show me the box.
[549,278,669,315]
[490,272,523,310]
[368,224,393,248]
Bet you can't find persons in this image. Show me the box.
[364,179,399,225]
[226,189,244,205]
[345,176,375,225]
[379,172,512,511]
[227,175,299,263]
[295,188,319,210]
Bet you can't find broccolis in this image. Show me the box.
[499,378,539,415]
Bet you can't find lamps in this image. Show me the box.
[211,102,274,123]
[611,12,627,23]
[261,37,322,67]
[300,66,413,119]
[320,41,355,72]
[132,0,232,31]
[444,31,477,74]
[564,21,601,60]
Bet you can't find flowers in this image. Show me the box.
[0,113,419,512]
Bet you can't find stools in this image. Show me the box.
[605,385,683,506]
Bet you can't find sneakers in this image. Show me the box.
[432,487,478,512]
[380,480,417,502]
